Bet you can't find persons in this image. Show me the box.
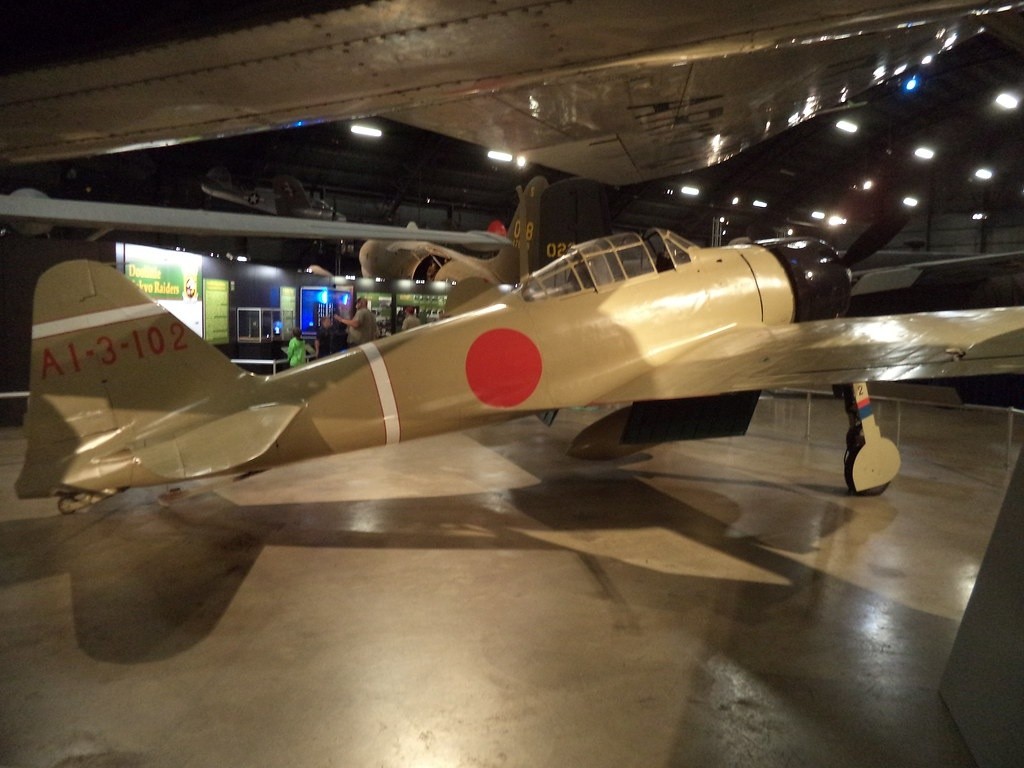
[334,298,376,348]
[315,316,348,360]
[402,306,421,331]
[288,327,315,368]
[397,310,405,332]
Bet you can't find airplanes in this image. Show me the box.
[0,174,1024,516]
